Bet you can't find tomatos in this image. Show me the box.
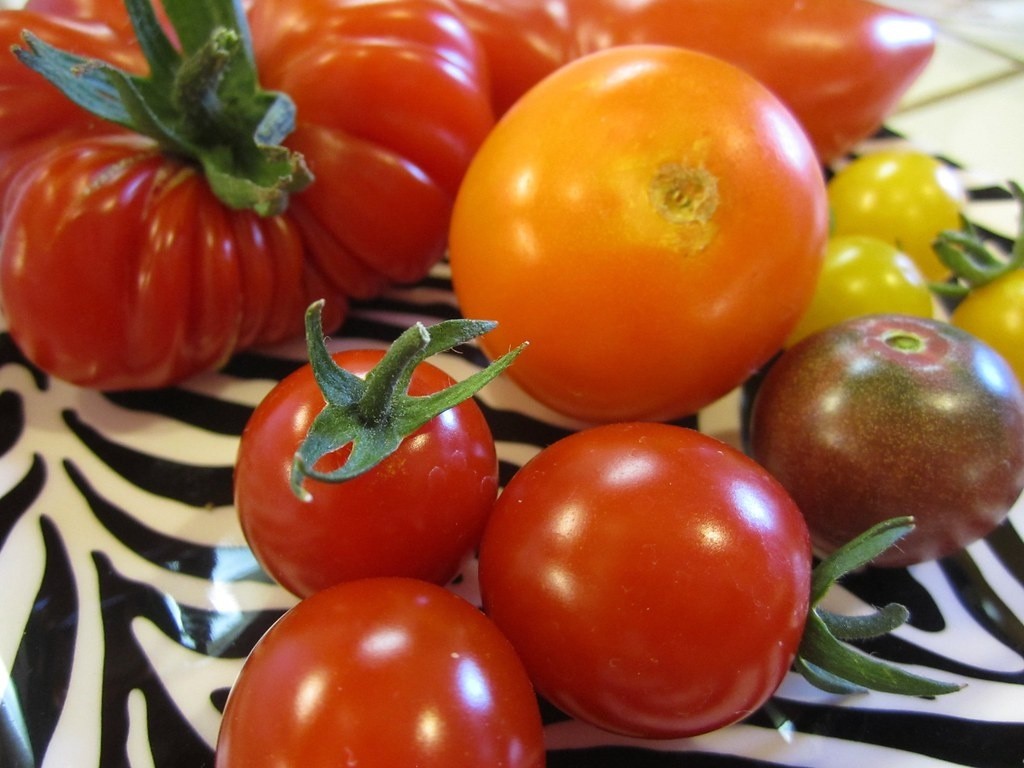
[0,2,1022,768]
